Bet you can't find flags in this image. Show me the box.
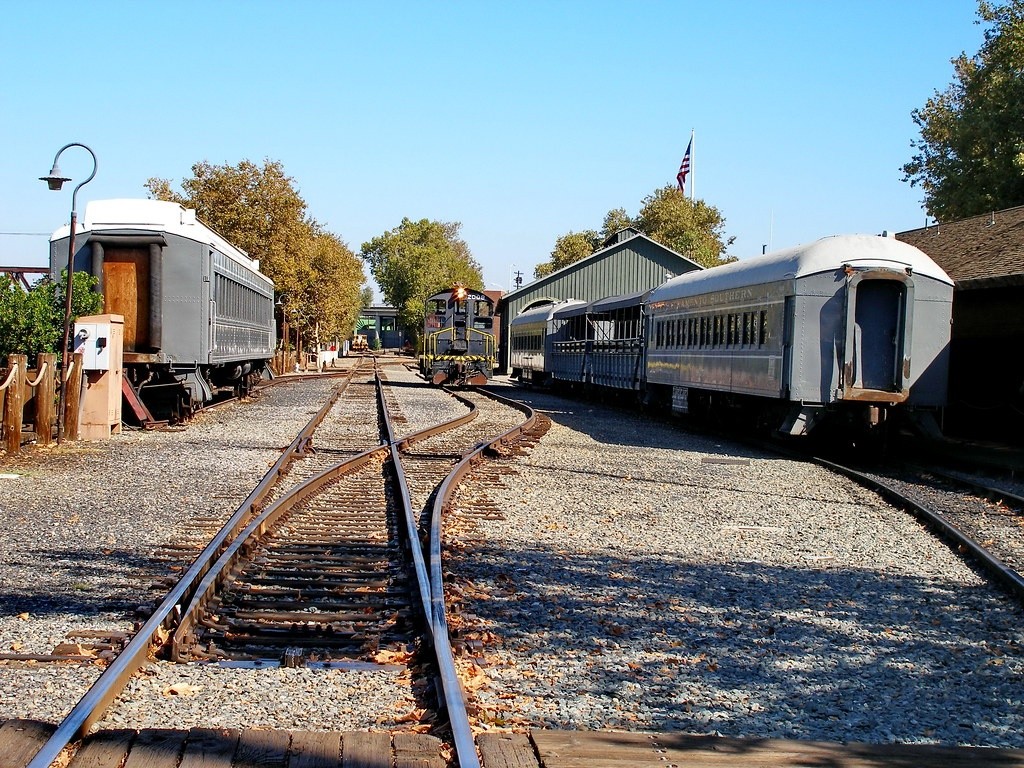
[676,135,693,195]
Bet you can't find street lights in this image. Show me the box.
[38,143,98,445]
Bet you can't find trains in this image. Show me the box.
[48,197,277,414]
[414,285,499,388]
[510,233,953,460]
